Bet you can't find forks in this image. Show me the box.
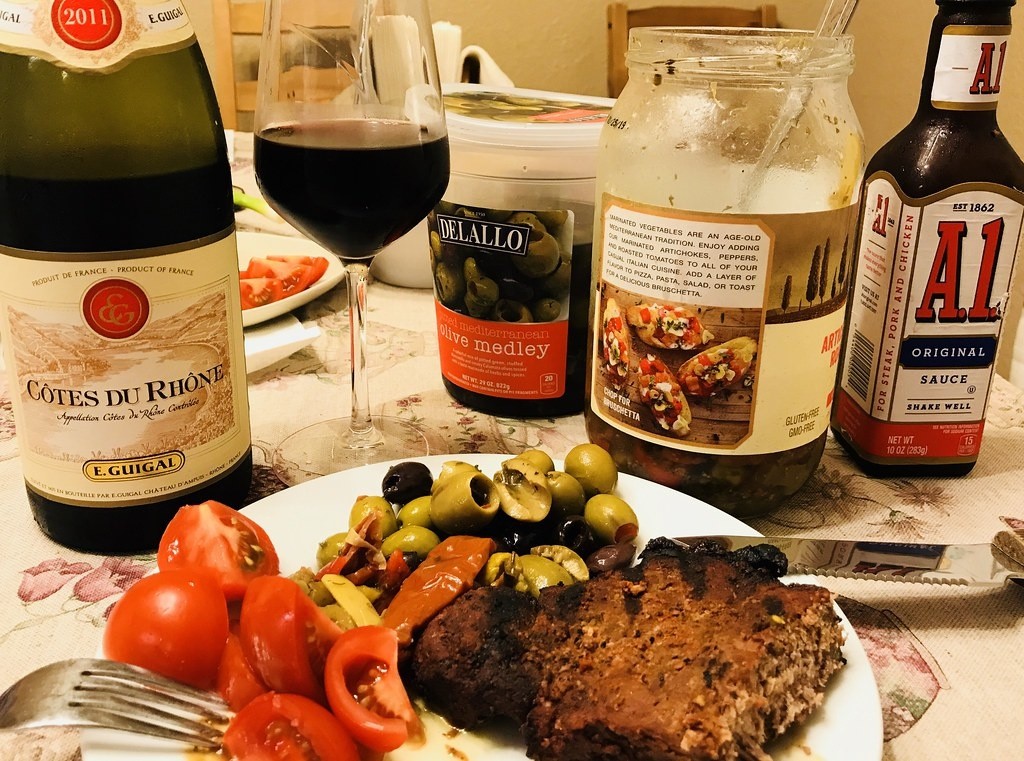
[0,659,228,749]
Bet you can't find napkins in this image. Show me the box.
[245,313,323,373]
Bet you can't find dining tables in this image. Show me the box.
[0,129,1024,761]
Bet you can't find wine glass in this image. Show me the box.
[253,1,451,485]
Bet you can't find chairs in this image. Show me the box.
[606,1,778,99]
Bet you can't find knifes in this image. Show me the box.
[675,537,1024,588]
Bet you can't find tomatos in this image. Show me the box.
[104,501,418,761]
[239,256,333,310]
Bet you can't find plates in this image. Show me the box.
[236,231,345,326]
[80,452,883,761]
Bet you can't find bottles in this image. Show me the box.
[831,0,1024,477]
[0,1,252,557]
[584,25,866,521]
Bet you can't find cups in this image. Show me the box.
[405,83,617,421]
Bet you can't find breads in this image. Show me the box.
[603,297,757,438]
